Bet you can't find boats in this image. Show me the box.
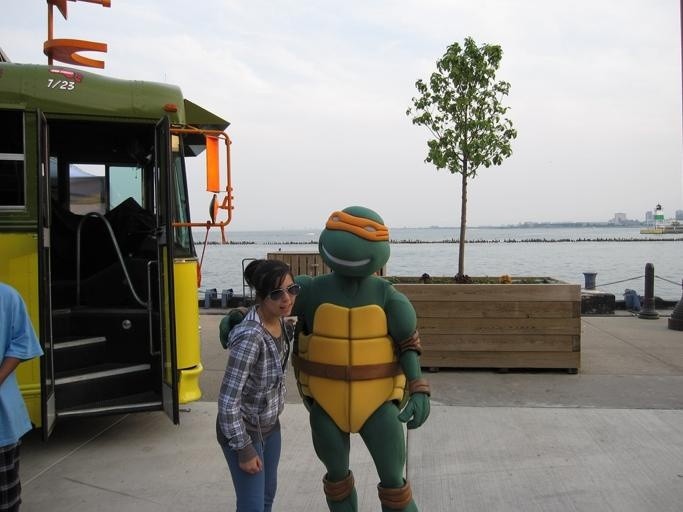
[654,220,682,234]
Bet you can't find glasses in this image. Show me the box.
[265,283,301,301]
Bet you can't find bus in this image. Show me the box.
[0,59,234,444]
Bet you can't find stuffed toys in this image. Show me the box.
[216,206,430,512]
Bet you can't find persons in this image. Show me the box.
[214,259,296,512]
[0,280,47,512]
